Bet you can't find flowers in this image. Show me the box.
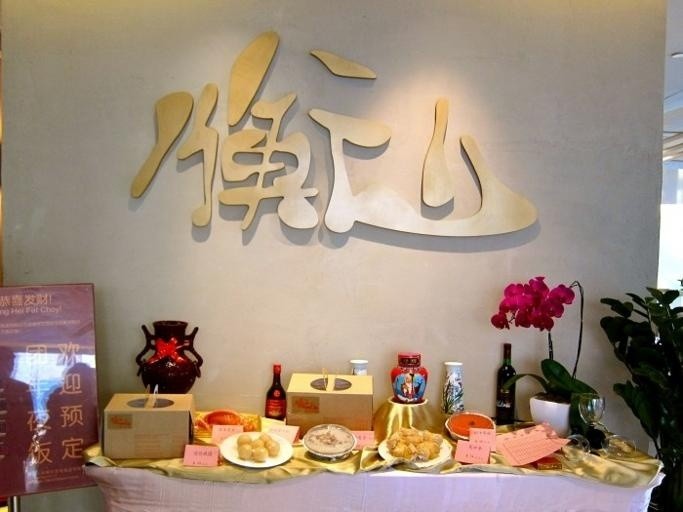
[489,276,605,437]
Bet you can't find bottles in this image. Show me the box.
[391,352,428,403]
[440,361,465,438]
[265,364,286,420]
[496,343,515,424]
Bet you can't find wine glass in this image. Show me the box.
[562,390,636,463]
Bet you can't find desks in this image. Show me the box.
[77,469,665,512]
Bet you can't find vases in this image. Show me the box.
[440,360,465,415]
[349,358,369,376]
[529,396,570,436]
[135,320,203,393]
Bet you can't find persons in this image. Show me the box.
[0,345,36,495]
[33,364,100,489]
[36,384,63,490]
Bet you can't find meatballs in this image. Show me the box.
[237,432,279,462]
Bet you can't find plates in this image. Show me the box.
[218,432,294,470]
[302,423,357,460]
[378,435,452,470]
